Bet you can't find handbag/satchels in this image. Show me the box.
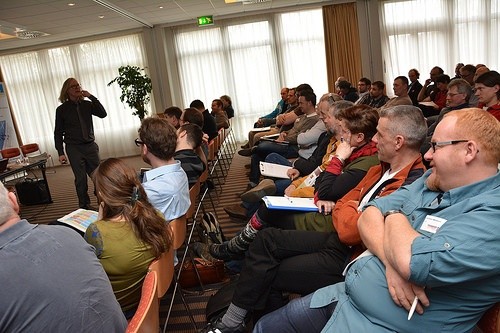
[174,258,224,287]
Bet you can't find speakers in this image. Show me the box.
[16,178,50,205]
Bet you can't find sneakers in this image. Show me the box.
[193,241,245,264]
[200,318,246,333]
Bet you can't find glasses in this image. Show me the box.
[135,138,151,150]
[328,93,335,102]
[358,84,365,86]
[66,84,82,90]
[295,94,301,96]
[446,93,462,98]
[429,140,479,155]
[459,73,471,78]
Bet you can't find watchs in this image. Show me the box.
[384,210,401,220]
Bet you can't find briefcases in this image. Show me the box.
[16,178,53,205]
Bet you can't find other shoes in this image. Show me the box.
[237,189,247,196]
[82,205,99,212]
[240,179,277,202]
[239,149,254,155]
[223,205,247,217]
[242,145,250,149]
[245,164,251,168]
[97,201,103,204]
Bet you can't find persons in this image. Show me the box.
[0,181,129,333]
[135,116,191,221]
[174,123,205,189]
[204,63,500,333]
[164,95,233,144]
[84,158,172,318]
[54,78,107,211]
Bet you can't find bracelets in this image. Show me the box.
[335,154,344,162]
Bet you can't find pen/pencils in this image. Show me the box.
[284,194,292,203]
[408,295,419,321]
[291,174,294,181]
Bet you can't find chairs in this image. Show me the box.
[0,148,29,180]
[125,118,238,333]
[20,143,56,178]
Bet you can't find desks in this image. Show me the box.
[0,159,47,179]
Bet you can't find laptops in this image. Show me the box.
[0,158,9,173]
